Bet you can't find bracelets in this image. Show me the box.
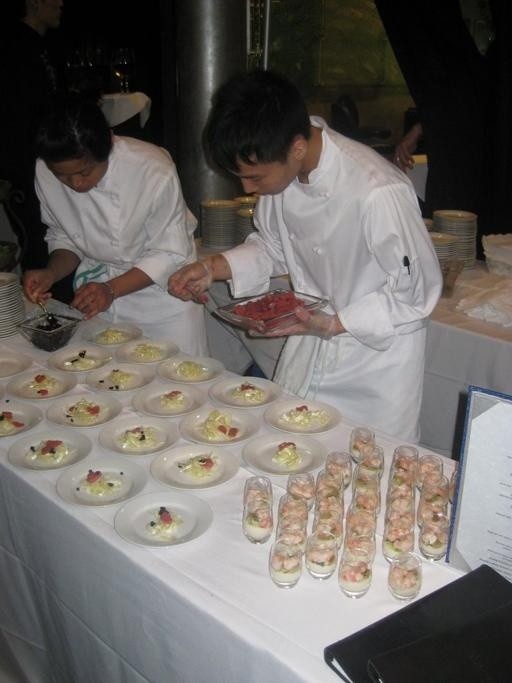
[104,282,114,301]
[201,262,211,285]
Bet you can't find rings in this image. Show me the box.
[395,157,399,162]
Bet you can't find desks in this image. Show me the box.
[193,236,512,462]
[1,290,512,683]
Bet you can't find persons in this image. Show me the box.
[394,123,423,174]
[166,72,444,445]
[0,0,104,270]
[23,89,209,357]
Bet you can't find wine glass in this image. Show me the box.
[62,42,136,93]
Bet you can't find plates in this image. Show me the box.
[150,443,240,488]
[86,361,155,393]
[179,405,260,445]
[98,416,183,457]
[5,427,93,471]
[46,392,123,427]
[214,287,329,333]
[46,344,114,372]
[207,376,283,408]
[263,399,341,436]
[0,272,34,339]
[114,490,214,546]
[110,337,179,364]
[242,434,329,475]
[199,196,259,250]
[84,323,144,345]
[154,354,224,385]
[0,401,43,440]
[5,369,78,401]
[55,459,150,507]
[132,382,208,418]
[1,349,34,378]
[421,209,478,276]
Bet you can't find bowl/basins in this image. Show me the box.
[16,311,83,351]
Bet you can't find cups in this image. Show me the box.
[242,427,460,601]
